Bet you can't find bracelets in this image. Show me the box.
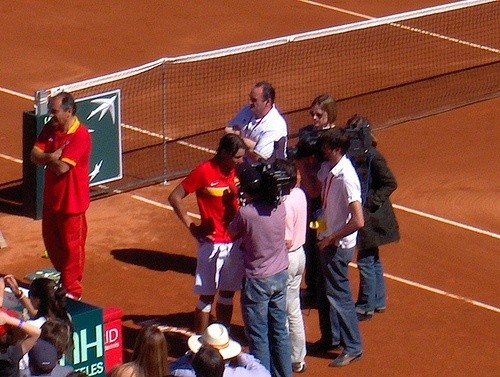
[17,320,23,329]
[16,289,25,301]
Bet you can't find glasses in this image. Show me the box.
[309,111,324,119]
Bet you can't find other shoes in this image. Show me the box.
[357,308,385,321]
[293,363,305,374]
[329,351,364,366]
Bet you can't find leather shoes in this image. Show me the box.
[308,339,340,354]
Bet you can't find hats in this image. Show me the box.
[188,323,241,360]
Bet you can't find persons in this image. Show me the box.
[0,274,271,377]
[167,81,401,377]
[31,91,91,300]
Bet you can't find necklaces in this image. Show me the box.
[245,106,273,138]
